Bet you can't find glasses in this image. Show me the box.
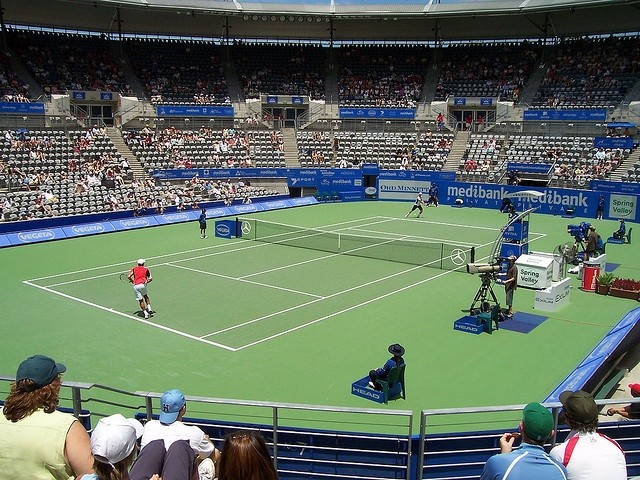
[57,373,64,380]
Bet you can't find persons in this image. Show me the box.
[481,402,568,480]
[436,111,445,128]
[335,46,431,109]
[0,164,68,220]
[476,115,484,132]
[585,224,597,259]
[199,209,208,237]
[68,153,130,211]
[436,43,541,108]
[127,114,259,172]
[131,174,253,213]
[127,260,156,321]
[219,430,283,479]
[606,383,639,426]
[406,192,424,218]
[543,144,619,182]
[612,219,626,241]
[465,115,473,133]
[1,355,97,479]
[4,128,57,161]
[605,128,630,140]
[369,344,405,388]
[71,412,215,479]
[497,255,517,316]
[297,130,451,169]
[462,134,496,176]
[537,35,640,110]
[427,182,439,209]
[132,43,234,107]
[500,206,517,243]
[548,390,628,480]
[0,40,132,102]
[140,389,221,478]
[242,46,326,103]
[75,123,107,153]
[271,132,284,153]
[596,234,604,249]
[596,194,607,220]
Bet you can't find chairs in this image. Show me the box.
[1,125,147,219]
[331,192,338,200]
[436,78,498,100]
[374,364,407,405]
[317,120,434,127]
[297,130,455,171]
[477,304,500,334]
[147,129,287,206]
[529,77,636,110]
[496,123,607,129]
[600,242,607,252]
[323,192,329,200]
[456,133,640,183]
[315,192,321,200]
[339,98,416,108]
[622,228,633,243]
[150,81,231,106]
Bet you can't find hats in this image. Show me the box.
[590,226,595,230]
[16,354,67,392]
[507,255,515,260]
[138,259,147,264]
[388,343,405,356]
[91,413,144,470]
[419,192,422,195]
[559,391,598,420]
[523,402,554,441]
[628,383,640,394]
[159,388,186,425]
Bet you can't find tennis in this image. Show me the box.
[426,206,428,208]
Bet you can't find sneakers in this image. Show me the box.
[511,312,514,317]
[198,457,215,480]
[145,313,150,319]
[369,381,376,388]
[149,310,154,317]
[507,314,511,318]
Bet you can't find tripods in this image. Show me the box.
[572,241,585,251]
[469,284,500,310]
